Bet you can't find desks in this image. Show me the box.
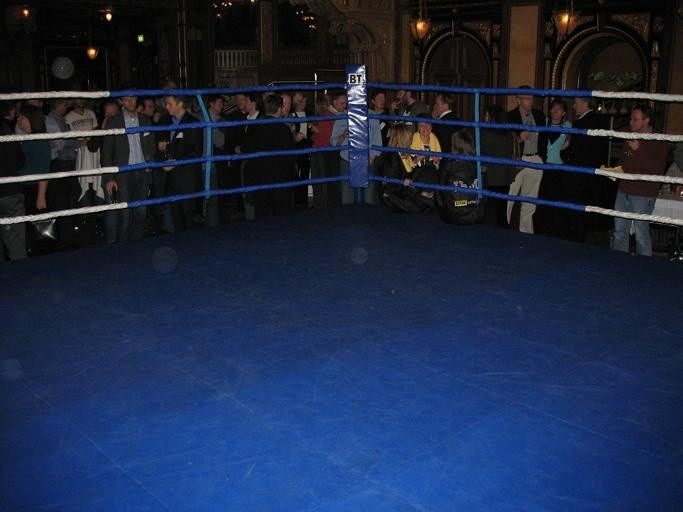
[647,181,682,258]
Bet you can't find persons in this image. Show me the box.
[0,86,682,261]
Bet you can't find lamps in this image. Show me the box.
[408,1,430,47]
[553,2,582,42]
